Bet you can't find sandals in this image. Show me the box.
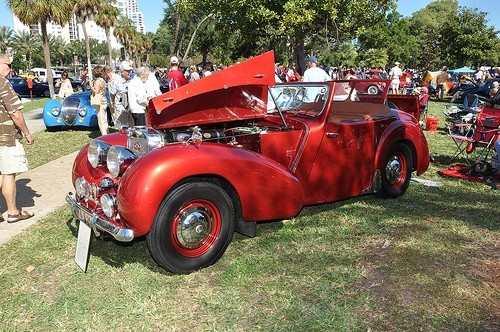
[0,212,5,222]
[7,210,35,223]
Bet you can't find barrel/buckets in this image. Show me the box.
[426,117,438,131]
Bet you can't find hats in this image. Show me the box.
[118,60,133,71]
[420,87,429,94]
[304,56,318,63]
[169,55,179,63]
[395,62,400,66]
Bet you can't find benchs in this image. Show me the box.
[300,101,390,122]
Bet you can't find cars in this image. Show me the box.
[42,88,112,132]
[342,69,388,95]
[7,67,96,98]
[461,77,500,106]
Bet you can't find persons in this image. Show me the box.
[0,54,35,224]
[58,73,74,100]
[90,55,500,136]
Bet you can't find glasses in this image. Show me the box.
[7,64,11,69]
[63,74,67,77]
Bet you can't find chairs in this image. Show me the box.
[429,84,439,100]
[10,80,15,83]
[21,80,25,83]
[446,104,500,168]
[444,94,478,136]
[419,95,431,120]
[19,81,23,84]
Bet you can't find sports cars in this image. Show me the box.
[65,47,429,275]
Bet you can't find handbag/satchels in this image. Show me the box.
[90,93,102,106]
